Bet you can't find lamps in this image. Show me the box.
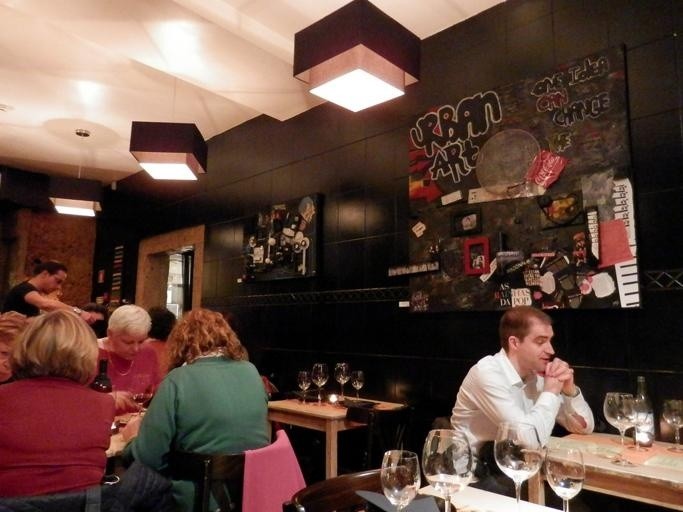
[293,0,420,113]
[47,128,104,217]
[128,80,209,181]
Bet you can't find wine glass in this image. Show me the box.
[297,371,311,405]
[544,438,584,512]
[351,370,363,403]
[379,450,421,512]
[662,399,682,453]
[420,429,472,512]
[603,392,637,466]
[333,363,350,402]
[130,373,153,420]
[310,363,329,406]
[493,422,543,511]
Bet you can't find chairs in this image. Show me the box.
[169,423,286,511]
[0,474,121,512]
[282,466,414,511]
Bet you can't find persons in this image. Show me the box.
[1,260,116,500]
[244,206,311,279]
[97,304,270,511]
[445,305,596,512]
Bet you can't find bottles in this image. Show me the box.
[94,269,108,305]
[633,376,654,448]
[89,359,112,394]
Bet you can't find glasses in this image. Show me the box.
[53,275,63,285]
